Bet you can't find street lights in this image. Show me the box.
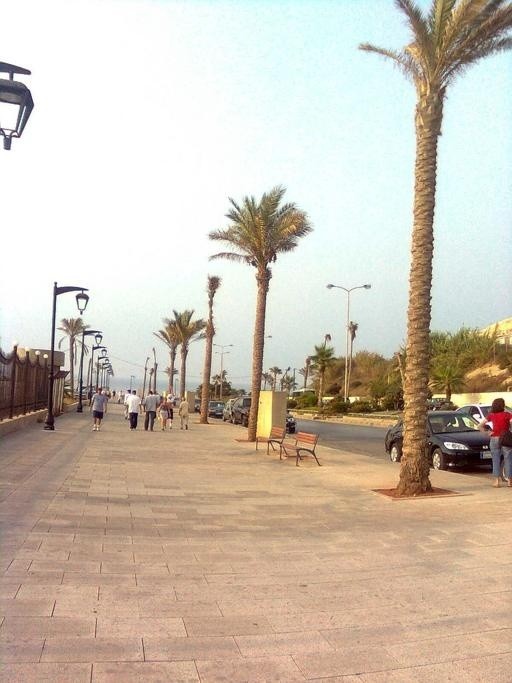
[0,58,36,152]
[33,350,41,411]
[42,353,49,409]
[213,343,234,400]
[23,344,31,415]
[76,327,102,412]
[43,281,91,430]
[326,282,372,406]
[90,344,115,412]
[8,339,20,420]
[265,334,272,339]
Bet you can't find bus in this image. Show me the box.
[291,387,317,400]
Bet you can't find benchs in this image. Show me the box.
[280,432,321,467]
[256,427,285,455]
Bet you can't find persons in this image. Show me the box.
[123,390,131,420]
[178,398,189,430]
[153,391,162,418]
[89,388,107,431]
[127,391,142,431]
[88,389,92,405]
[166,393,180,429]
[158,398,171,430]
[143,391,157,431]
[480,397,512,488]
[102,390,125,403]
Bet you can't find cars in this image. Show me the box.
[426,397,452,411]
[454,403,511,438]
[384,408,503,471]
[173,396,296,434]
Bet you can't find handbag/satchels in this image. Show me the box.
[499,431,512,447]
